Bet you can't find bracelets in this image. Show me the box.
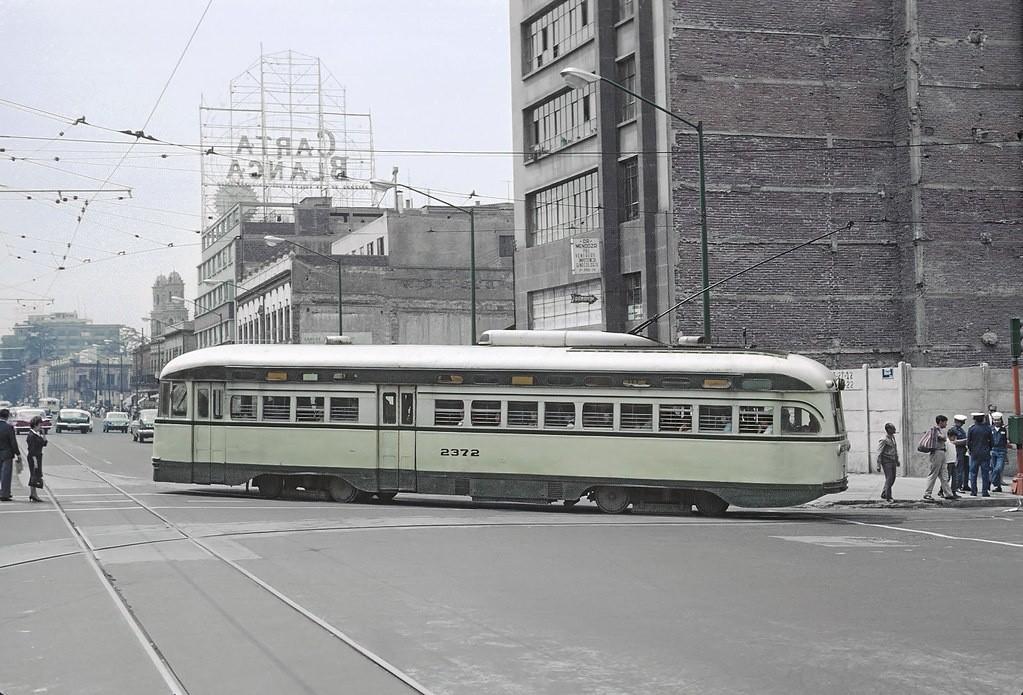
[17,455,21,458]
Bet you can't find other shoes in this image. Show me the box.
[988,479,991,491]
[881,495,887,499]
[970,492,976,496]
[963,486,971,491]
[937,491,945,498]
[993,486,1001,492]
[924,494,935,500]
[983,492,990,497]
[0,494,12,500]
[888,498,894,503]
[953,493,961,498]
[955,489,965,493]
[1,497,12,501]
[945,496,958,500]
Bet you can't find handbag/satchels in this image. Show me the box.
[918,427,941,453]
[28,468,44,489]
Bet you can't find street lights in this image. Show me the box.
[74,350,111,412]
[203,279,266,345]
[369,180,476,345]
[104,339,139,403]
[560,66,710,343]
[92,344,124,395]
[121,327,160,383]
[171,296,222,345]
[263,236,343,336]
[142,318,185,354]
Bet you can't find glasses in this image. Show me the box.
[989,409,996,412]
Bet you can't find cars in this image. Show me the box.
[56,409,91,433]
[85,411,93,432]
[103,412,129,433]
[43,408,52,418]
[130,409,158,443]
[9,409,53,435]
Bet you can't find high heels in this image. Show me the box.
[29,495,42,502]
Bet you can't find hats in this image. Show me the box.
[954,414,967,424]
[992,412,1003,419]
[29,415,41,427]
[971,412,985,419]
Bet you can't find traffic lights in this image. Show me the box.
[1019,320,1023,353]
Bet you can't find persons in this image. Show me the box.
[989,412,1008,493]
[948,414,971,494]
[967,412,993,497]
[26,416,48,503]
[877,423,901,504]
[762,407,794,436]
[453,401,733,432]
[923,415,958,501]
[937,428,961,499]
[0,408,22,502]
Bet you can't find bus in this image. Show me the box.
[151,328,851,517]
[39,398,60,414]
